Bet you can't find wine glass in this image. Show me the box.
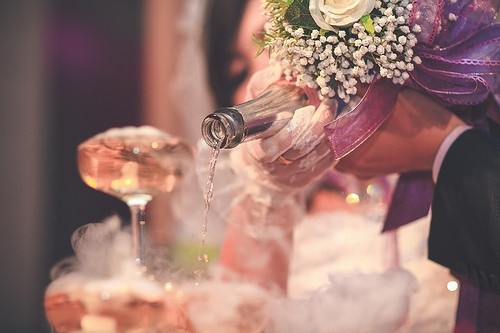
[79,136,189,294]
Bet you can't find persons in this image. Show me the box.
[334,85,500,333]
[206,1,353,215]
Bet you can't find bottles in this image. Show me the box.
[201,76,324,151]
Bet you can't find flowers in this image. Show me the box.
[250,0,500,161]
